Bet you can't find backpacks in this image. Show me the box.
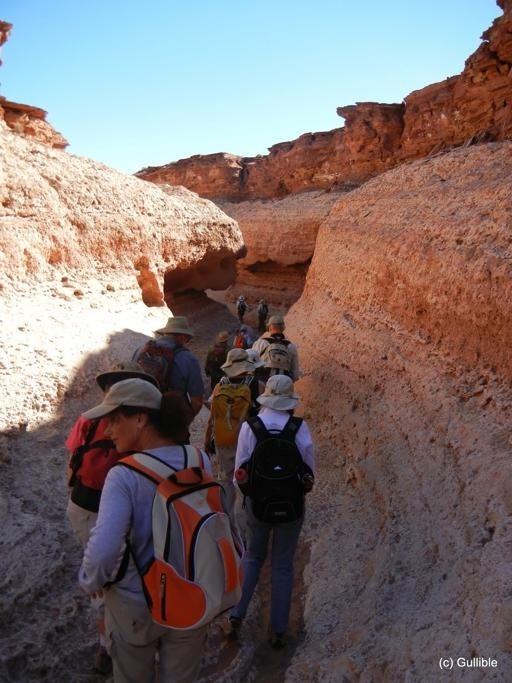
[237,417,314,523]
[210,378,254,448]
[257,334,291,378]
[116,445,245,630]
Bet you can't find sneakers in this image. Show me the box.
[94,650,112,674]
[219,620,240,638]
[267,624,286,649]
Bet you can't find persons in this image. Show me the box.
[67,360,162,669]
[228,374,317,648]
[206,295,301,510]
[78,377,215,683]
[133,316,206,446]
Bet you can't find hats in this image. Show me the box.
[256,374,302,411]
[236,295,285,327]
[83,362,164,420]
[155,316,264,377]
